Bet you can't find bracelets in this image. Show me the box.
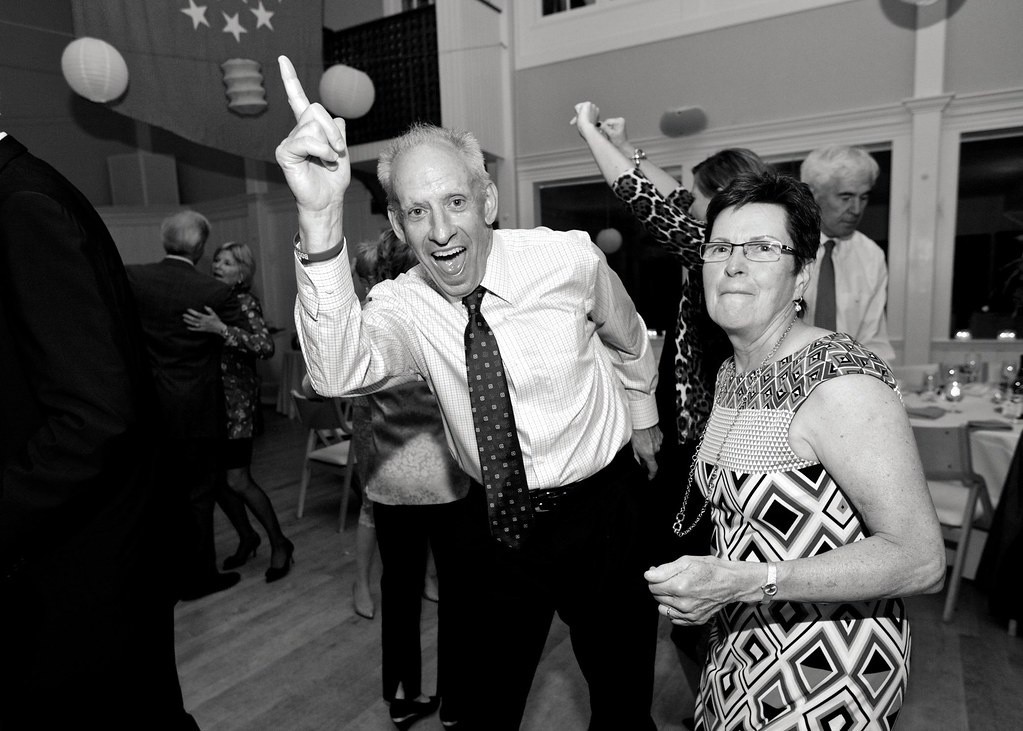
[219,327,229,337]
[295,237,348,266]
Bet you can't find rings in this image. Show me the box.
[665,607,672,617]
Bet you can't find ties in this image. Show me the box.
[814,240,836,332]
[462,285,536,551]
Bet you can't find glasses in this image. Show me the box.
[700,241,810,262]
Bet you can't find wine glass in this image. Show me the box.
[923,353,1022,413]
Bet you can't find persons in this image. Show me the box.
[274,52,663,731]
[123,209,296,603]
[0,132,198,731]
[644,170,945,731]
[567,98,895,694]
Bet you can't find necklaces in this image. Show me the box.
[672,315,801,540]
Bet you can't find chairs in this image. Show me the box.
[286,390,362,530]
[913,423,994,623]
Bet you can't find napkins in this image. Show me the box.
[906,405,944,419]
[965,417,1012,432]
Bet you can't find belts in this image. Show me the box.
[470,461,623,514]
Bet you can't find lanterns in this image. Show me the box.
[219,57,269,116]
[60,35,130,104]
[318,62,375,119]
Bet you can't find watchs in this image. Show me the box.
[762,562,779,609]
[632,145,648,161]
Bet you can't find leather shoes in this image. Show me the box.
[424,585,439,602]
[353,582,375,617]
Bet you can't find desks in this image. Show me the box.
[901,390,1023,524]
[277,349,311,420]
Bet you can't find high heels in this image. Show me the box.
[265,539,294,580]
[389,697,440,731]
[223,534,261,569]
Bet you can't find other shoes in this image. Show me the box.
[178,573,241,601]
[442,718,459,731]
